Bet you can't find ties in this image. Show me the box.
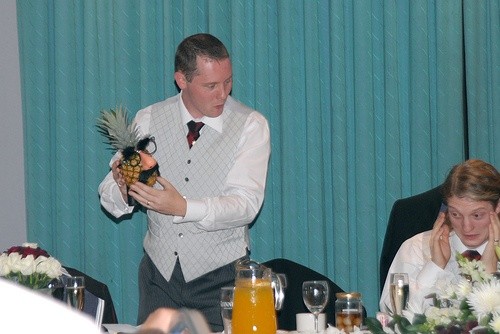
[187,120,205,148]
[457,250,481,282]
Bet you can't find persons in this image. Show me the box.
[97,33,272,334]
[379,158,500,314]
[0,276,179,334]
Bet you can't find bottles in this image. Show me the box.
[335,293,363,334]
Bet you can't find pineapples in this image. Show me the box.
[96,105,158,187]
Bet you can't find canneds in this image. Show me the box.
[334,292,363,333]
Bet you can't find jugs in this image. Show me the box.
[232,259,285,334]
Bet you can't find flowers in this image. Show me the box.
[0,242,70,287]
[363,249,500,334]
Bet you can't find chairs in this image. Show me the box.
[380,184,452,306]
[264,258,370,331]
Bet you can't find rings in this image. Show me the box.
[147,200,150,205]
[494,239,499,242]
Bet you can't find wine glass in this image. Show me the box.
[302,280,329,334]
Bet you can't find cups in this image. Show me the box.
[390,273,410,316]
[53,276,85,313]
[221,286,234,334]
[296,313,326,334]
